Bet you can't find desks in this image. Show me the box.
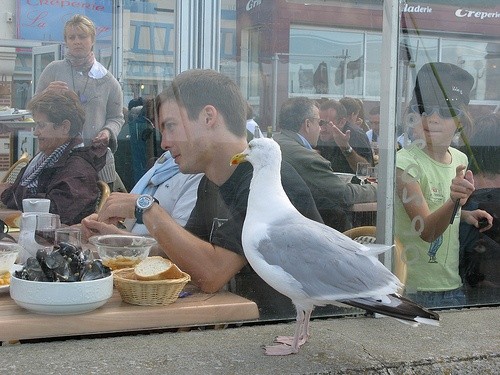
[334,172,378,230]
[0,204,259,341]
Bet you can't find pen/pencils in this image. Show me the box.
[449,159,472,224]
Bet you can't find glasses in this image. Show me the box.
[369,121,380,125]
[411,105,460,117]
[312,117,324,126]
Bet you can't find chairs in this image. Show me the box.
[342,226,408,289]
[2,158,28,183]
[97,179,110,212]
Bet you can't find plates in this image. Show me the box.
[0,110,23,120]
[0,121,35,128]
[13,110,32,114]
[0,267,10,294]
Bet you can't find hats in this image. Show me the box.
[416,62,474,105]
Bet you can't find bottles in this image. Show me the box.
[267,126,273,138]
[254,126,260,138]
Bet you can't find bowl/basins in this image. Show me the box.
[86,234,158,271]
[9,264,114,316]
[334,172,355,183]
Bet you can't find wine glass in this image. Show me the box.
[367,167,378,185]
[356,162,370,185]
[34,214,61,255]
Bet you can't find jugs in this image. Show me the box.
[21,213,51,231]
[23,198,50,213]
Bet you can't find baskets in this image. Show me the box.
[113,268,191,306]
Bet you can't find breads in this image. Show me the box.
[125,256,185,280]
[4,213,21,228]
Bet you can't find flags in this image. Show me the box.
[313,55,363,91]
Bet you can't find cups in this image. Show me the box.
[55,228,82,245]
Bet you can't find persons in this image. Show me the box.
[81,69,326,319]
[394,61,492,308]
[458,111,500,307]
[33,15,125,191]
[116,97,162,186]
[0,81,98,225]
[243,91,378,231]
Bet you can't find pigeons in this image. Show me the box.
[230,137,441,355]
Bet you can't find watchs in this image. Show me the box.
[134,193,160,225]
